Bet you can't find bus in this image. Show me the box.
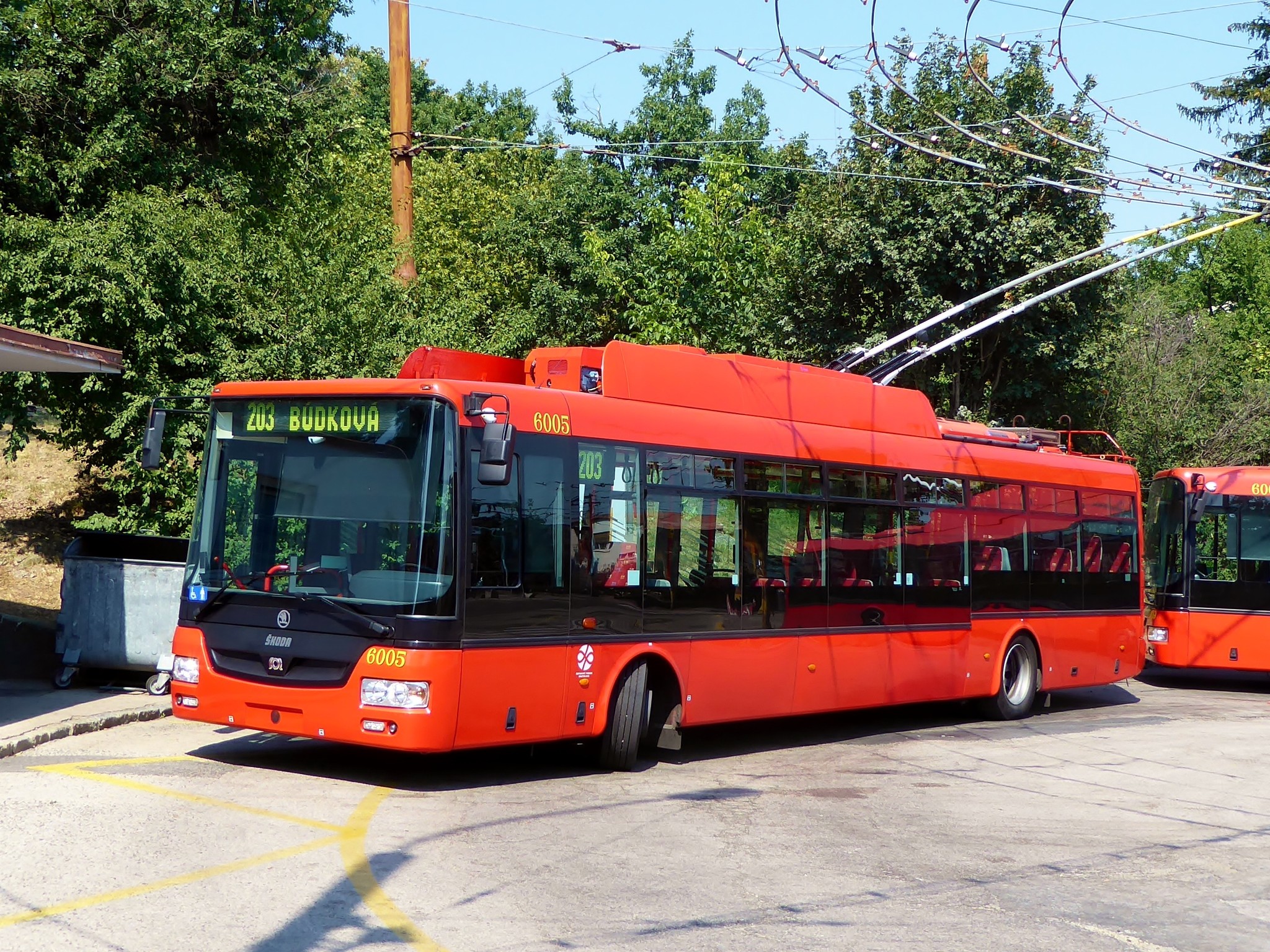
[140,340,1152,768]
[1140,465,1270,684]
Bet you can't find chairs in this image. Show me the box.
[751,535,1130,587]
